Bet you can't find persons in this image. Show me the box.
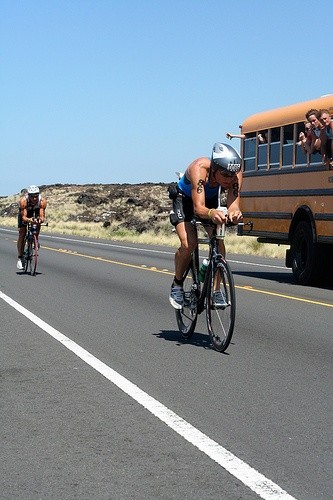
[226,132,247,141]
[17,185,47,270]
[299,121,316,155]
[314,109,333,166]
[168,142,243,311]
[258,131,273,144]
[306,109,325,154]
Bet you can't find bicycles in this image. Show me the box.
[22,216,49,276]
[175,207,254,352]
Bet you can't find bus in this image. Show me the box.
[226,94,333,282]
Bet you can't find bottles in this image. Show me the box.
[217,206,228,240]
[197,257,209,283]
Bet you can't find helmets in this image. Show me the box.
[27,185,40,195]
[211,142,242,175]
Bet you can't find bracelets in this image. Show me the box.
[208,209,214,219]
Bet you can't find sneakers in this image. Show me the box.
[211,289,228,307]
[168,283,185,310]
[16,260,25,270]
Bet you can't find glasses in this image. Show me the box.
[217,170,237,178]
